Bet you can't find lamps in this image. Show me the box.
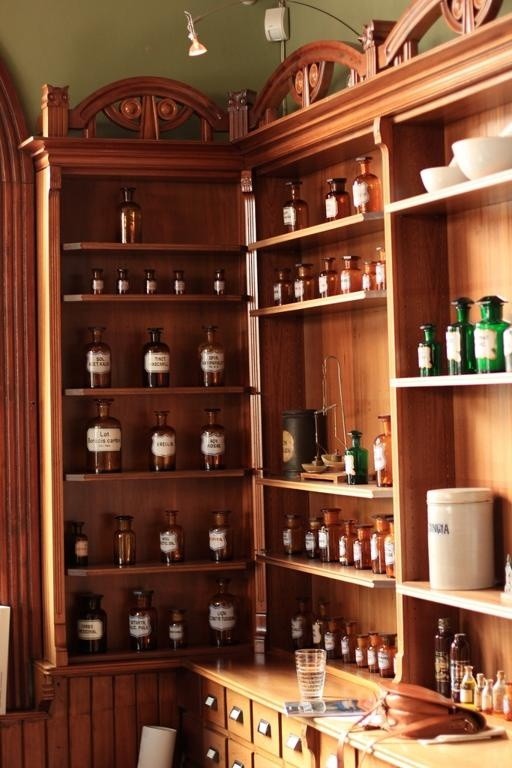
[184,0,362,57]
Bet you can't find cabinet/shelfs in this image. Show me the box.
[248,71,399,695]
[377,15,511,729]
[185,665,511,768]
[16,131,257,676]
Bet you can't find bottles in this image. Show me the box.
[92,267,104,295]
[433,618,512,720]
[157,509,185,567]
[74,593,106,655]
[117,267,128,296]
[197,325,225,390]
[84,399,123,475]
[206,507,229,564]
[68,521,89,568]
[165,607,187,652]
[282,180,309,232]
[342,430,369,485]
[197,407,226,469]
[145,267,157,295]
[148,410,178,474]
[82,324,113,389]
[205,580,240,646]
[353,157,380,214]
[291,595,396,679]
[124,589,156,653]
[283,507,396,577]
[273,247,388,305]
[372,416,392,487]
[118,186,143,244]
[475,295,509,374]
[114,514,137,568]
[322,178,351,222]
[172,267,186,296]
[444,297,473,375]
[417,323,441,376]
[140,326,170,389]
[210,269,227,297]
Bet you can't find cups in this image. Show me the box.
[295,648,326,701]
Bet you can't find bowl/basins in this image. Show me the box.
[450,137,511,180]
[419,166,470,192]
